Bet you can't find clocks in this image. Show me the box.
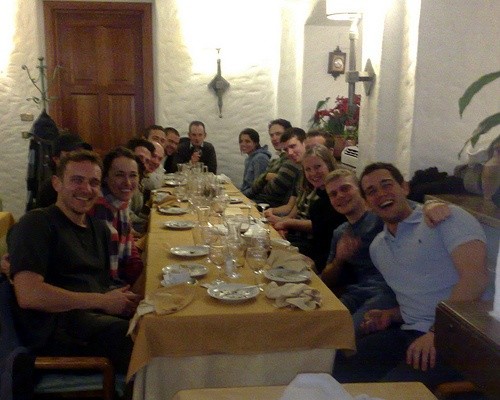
[328,46,346,80]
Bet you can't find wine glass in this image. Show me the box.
[208,239,228,285]
[245,202,269,229]
[244,226,272,294]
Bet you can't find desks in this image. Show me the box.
[424,195,500,302]
[434,300,500,364]
[125,170,355,400]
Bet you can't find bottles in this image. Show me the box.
[223,220,247,279]
[192,206,214,246]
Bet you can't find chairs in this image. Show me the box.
[0,271,129,400]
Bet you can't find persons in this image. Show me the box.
[6,148,140,400]
[236,119,449,383]
[162,121,217,177]
[357,161,488,383]
[85,124,180,301]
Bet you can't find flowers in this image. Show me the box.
[308,94,361,139]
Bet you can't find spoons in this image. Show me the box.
[186,279,196,285]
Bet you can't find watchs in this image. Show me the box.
[429,326,436,333]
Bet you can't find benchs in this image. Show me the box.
[172,382,438,400]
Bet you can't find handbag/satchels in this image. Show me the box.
[407,167,464,204]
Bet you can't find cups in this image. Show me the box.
[177,164,186,175]
[196,162,208,174]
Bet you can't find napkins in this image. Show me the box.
[266,248,314,274]
[126,283,196,342]
[266,281,323,311]
[154,194,180,211]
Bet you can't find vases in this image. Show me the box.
[333,135,357,161]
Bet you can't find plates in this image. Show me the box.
[160,278,199,287]
[207,283,260,302]
[264,269,311,283]
[161,263,208,277]
[169,246,210,257]
[164,221,195,228]
[165,180,186,185]
[220,197,242,203]
[159,207,186,215]
